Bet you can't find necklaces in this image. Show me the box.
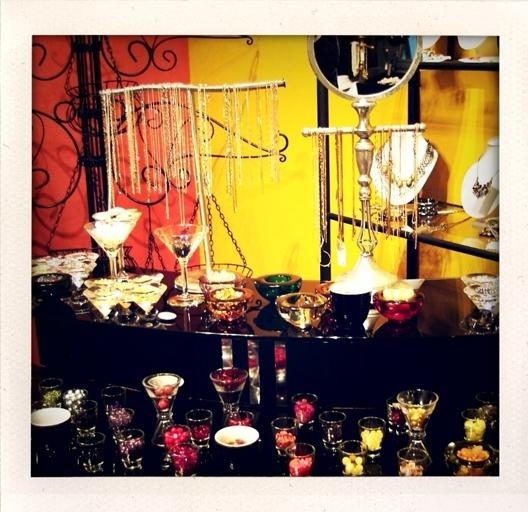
[97,77,284,228]
[472,162,497,199]
[312,123,436,270]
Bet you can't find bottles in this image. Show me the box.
[185,409,214,449]
[209,366,249,413]
[226,403,251,426]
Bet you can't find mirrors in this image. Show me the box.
[307,35,424,257]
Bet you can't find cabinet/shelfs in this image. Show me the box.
[316,61,499,284]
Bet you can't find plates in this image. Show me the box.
[363,279,425,318]
[173,273,203,295]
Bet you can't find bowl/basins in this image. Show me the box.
[214,426,260,450]
[373,290,425,323]
[453,440,491,467]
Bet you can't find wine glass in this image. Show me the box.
[83,207,142,284]
[153,224,209,307]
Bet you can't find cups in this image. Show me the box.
[318,411,346,450]
[459,307,499,336]
[199,271,243,300]
[31,252,99,313]
[141,373,184,447]
[253,273,303,300]
[387,397,407,434]
[459,270,498,311]
[330,285,372,328]
[31,375,146,479]
[396,389,439,439]
[204,287,253,320]
[81,273,167,329]
[284,443,316,477]
[161,424,198,477]
[358,417,387,462]
[271,417,299,457]
[275,291,328,329]
[292,392,318,431]
[397,447,427,476]
[338,440,367,476]
[461,408,486,441]
